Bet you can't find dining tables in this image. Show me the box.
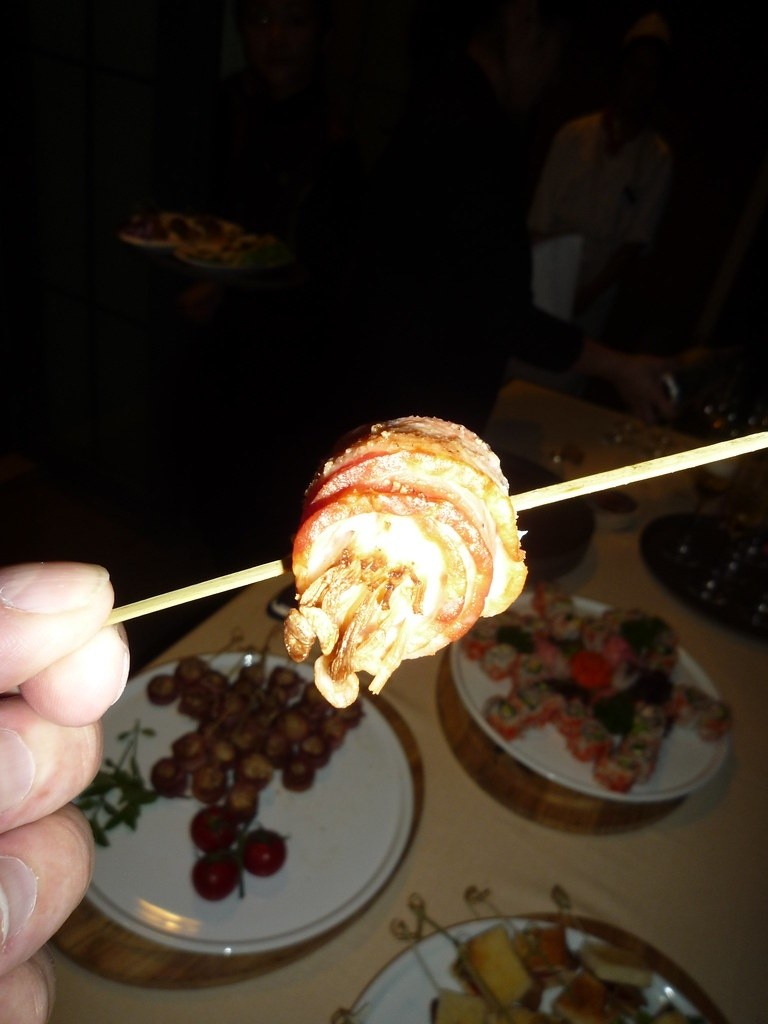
[40,381,767,1024]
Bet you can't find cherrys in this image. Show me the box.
[148,653,361,817]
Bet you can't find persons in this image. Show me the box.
[138,0,746,547]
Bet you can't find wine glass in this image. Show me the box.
[681,421,768,620]
[604,369,680,449]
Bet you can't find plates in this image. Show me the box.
[450,592,731,801]
[72,652,415,957]
[346,915,708,1023]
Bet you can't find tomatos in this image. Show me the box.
[189,804,286,900]
